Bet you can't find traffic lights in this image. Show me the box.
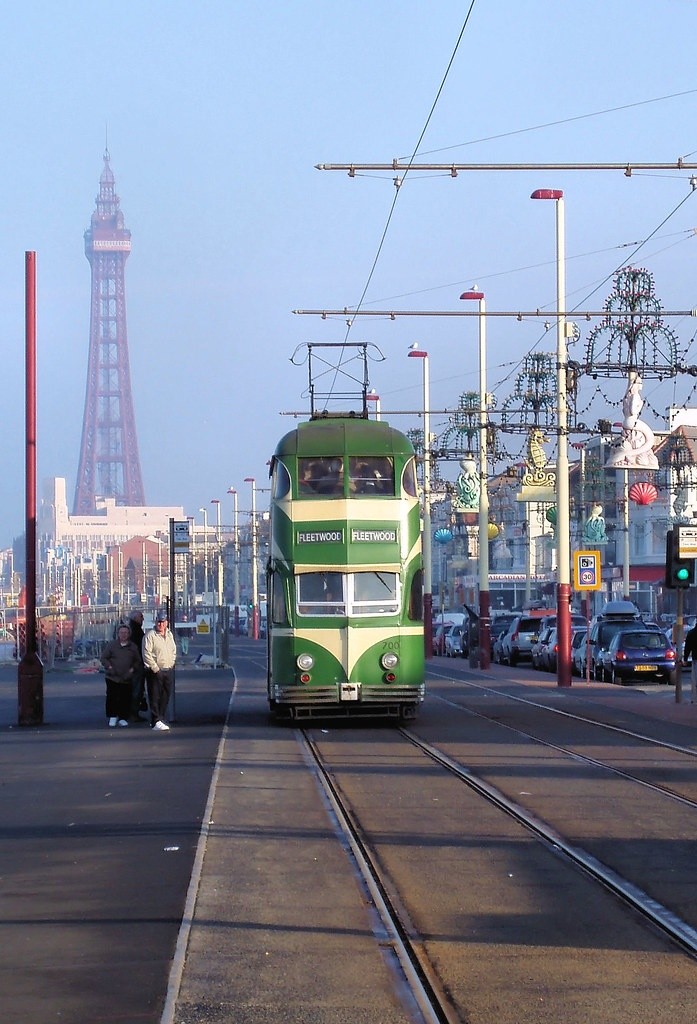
[665,529,693,589]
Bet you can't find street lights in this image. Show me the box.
[198,507,209,614]
[530,188,574,687]
[186,517,197,632]
[226,490,239,637]
[613,422,632,600]
[243,476,259,641]
[570,442,588,619]
[513,463,532,611]
[459,291,492,671]
[405,350,434,663]
[210,499,224,606]
[364,394,381,421]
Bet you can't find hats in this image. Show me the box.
[156,613,167,623]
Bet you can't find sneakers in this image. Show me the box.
[139,715,148,722]
[185,654,188,656]
[117,720,128,727]
[182,652,185,655]
[109,718,117,726]
[129,716,139,723]
[152,721,170,730]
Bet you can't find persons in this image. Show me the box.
[129,610,147,723]
[297,456,415,498]
[178,614,194,656]
[300,572,399,614]
[682,620,697,704]
[622,367,643,417]
[142,613,176,730]
[100,624,141,727]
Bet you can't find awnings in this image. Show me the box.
[629,567,666,584]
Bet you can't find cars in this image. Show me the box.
[431,600,697,686]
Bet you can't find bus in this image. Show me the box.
[263,341,428,723]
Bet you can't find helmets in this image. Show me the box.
[181,614,188,621]
